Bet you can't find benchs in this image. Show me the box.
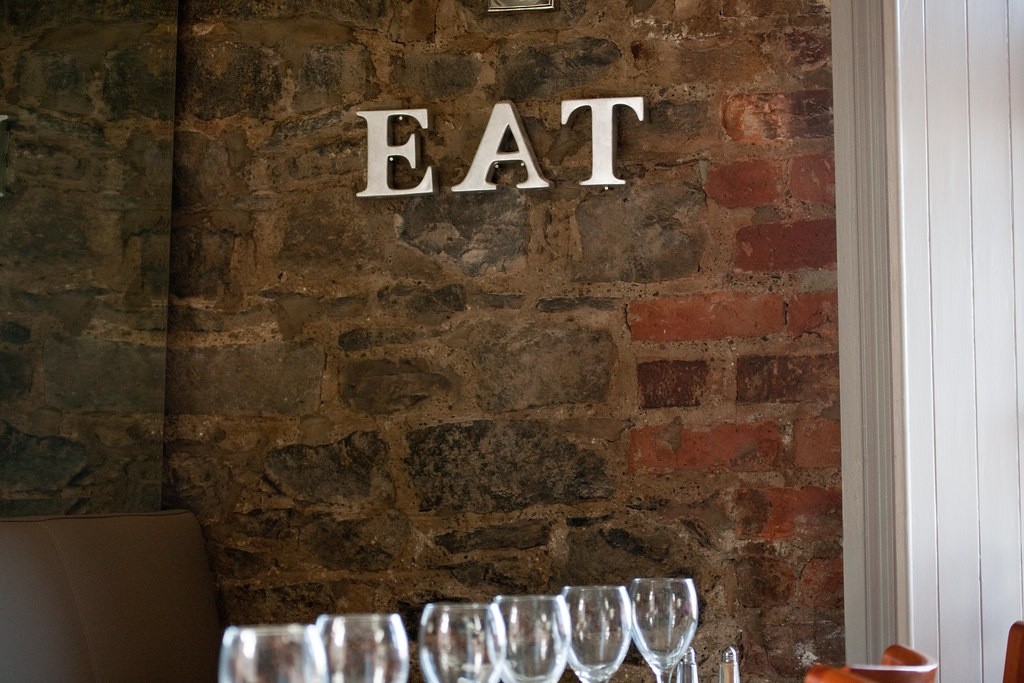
[0,509,224,683]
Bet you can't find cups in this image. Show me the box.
[216,623,329,683]
[418,601,508,682]
[486,594,574,683]
[314,612,410,683]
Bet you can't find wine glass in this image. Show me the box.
[557,586,634,683]
[629,576,698,683]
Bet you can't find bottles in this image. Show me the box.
[676,645,698,683]
[718,647,740,683]
